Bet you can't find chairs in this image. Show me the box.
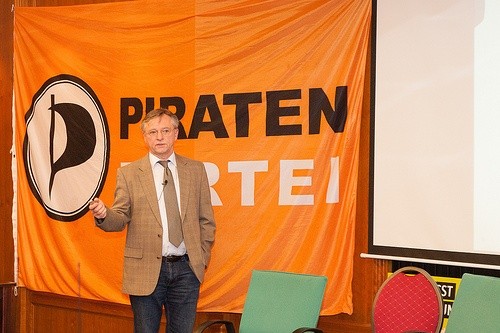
[371,267,444,333]
[194,270,328,333]
[445,273,500,333]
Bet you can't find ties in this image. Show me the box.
[157,160,183,248]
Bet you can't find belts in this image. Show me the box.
[162,255,188,263]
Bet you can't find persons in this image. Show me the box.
[88,109,216,333]
